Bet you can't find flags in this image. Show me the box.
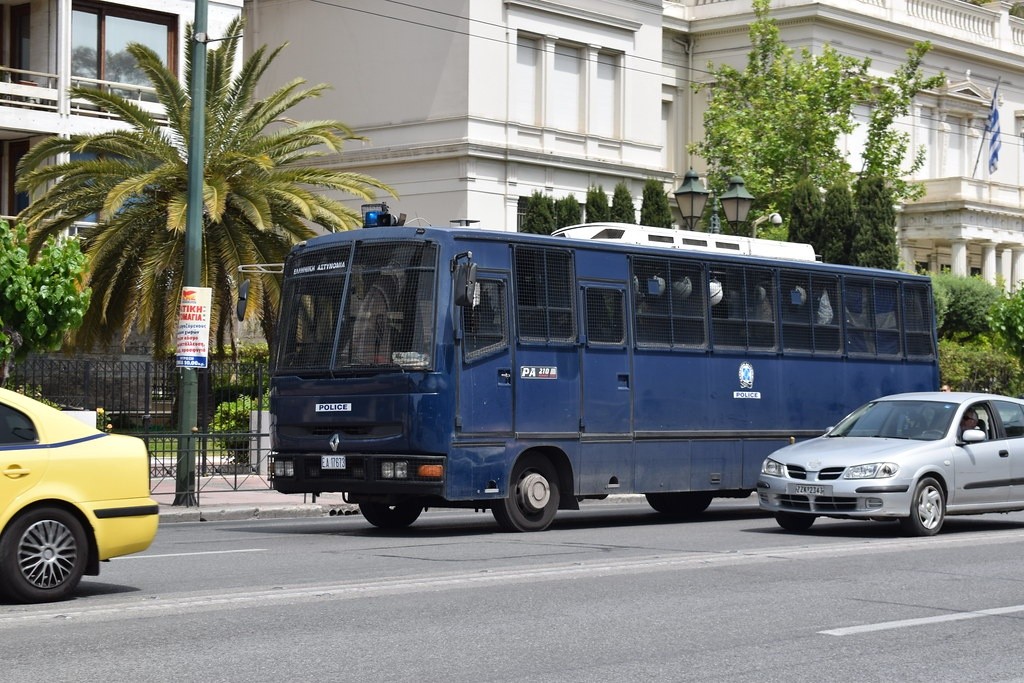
[986,92,1001,173]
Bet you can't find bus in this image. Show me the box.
[235,198,943,535]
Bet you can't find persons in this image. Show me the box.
[960,409,987,441]
[941,385,951,392]
[342,319,382,361]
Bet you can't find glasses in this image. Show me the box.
[963,416,975,421]
[941,390,946,392]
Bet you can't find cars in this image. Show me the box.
[756,391,1024,536]
[0,386,160,604]
